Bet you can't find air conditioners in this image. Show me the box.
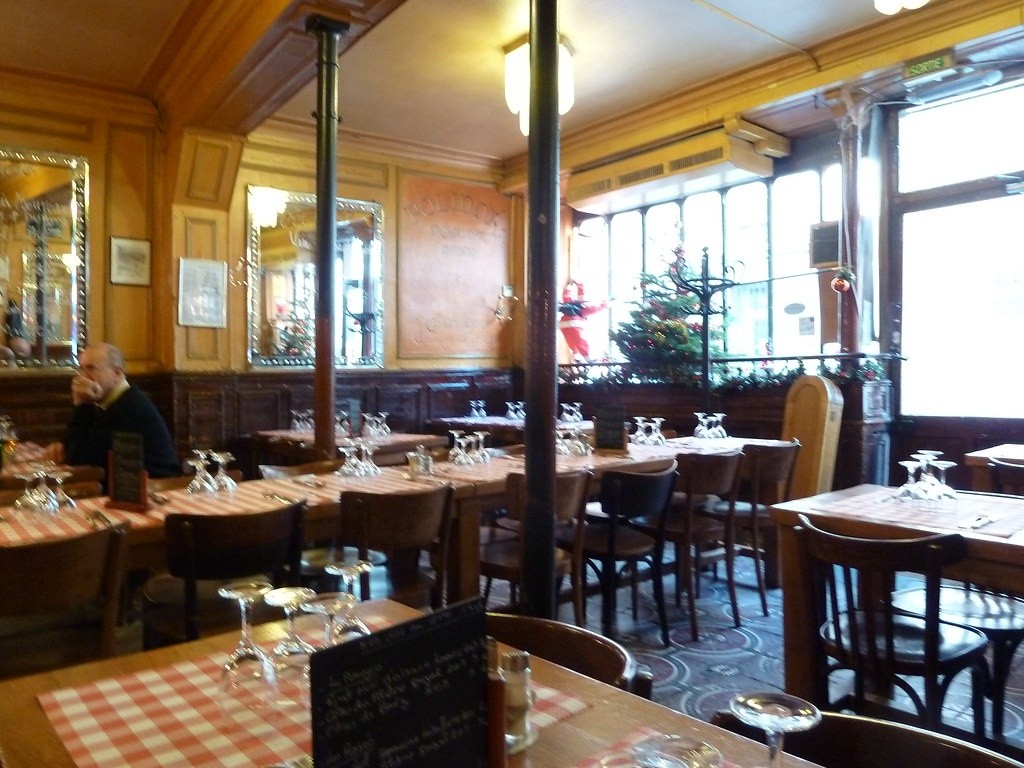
[562,119,775,214]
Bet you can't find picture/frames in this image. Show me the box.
[110,235,151,286]
[179,256,231,327]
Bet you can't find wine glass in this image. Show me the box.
[298,590,361,711]
[693,412,728,440]
[633,416,666,450]
[361,412,391,441]
[325,559,374,645]
[729,692,822,767]
[264,585,318,712]
[218,582,276,712]
[290,409,315,435]
[338,442,381,479]
[468,400,487,419]
[560,402,583,424]
[334,410,350,439]
[8,456,76,518]
[506,401,526,421]
[892,449,960,515]
[186,449,238,498]
[448,429,491,468]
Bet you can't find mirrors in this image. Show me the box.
[0,146,95,373]
[244,185,387,369]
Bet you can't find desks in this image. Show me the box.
[773,484,1024,693]
[963,442,1024,495]
[1,599,823,768]
[0,416,801,545]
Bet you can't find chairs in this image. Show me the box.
[338,483,456,611]
[480,612,656,700]
[987,456,1024,496]
[593,460,677,647]
[892,587,1024,746]
[165,499,308,644]
[747,438,800,617]
[709,706,1024,768]
[679,448,742,640]
[483,472,590,627]
[795,508,988,741]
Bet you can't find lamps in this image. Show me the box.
[502,28,581,138]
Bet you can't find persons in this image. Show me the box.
[1,338,39,359]
[559,280,611,364]
[43,342,183,480]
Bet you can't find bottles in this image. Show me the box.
[497,648,532,756]
